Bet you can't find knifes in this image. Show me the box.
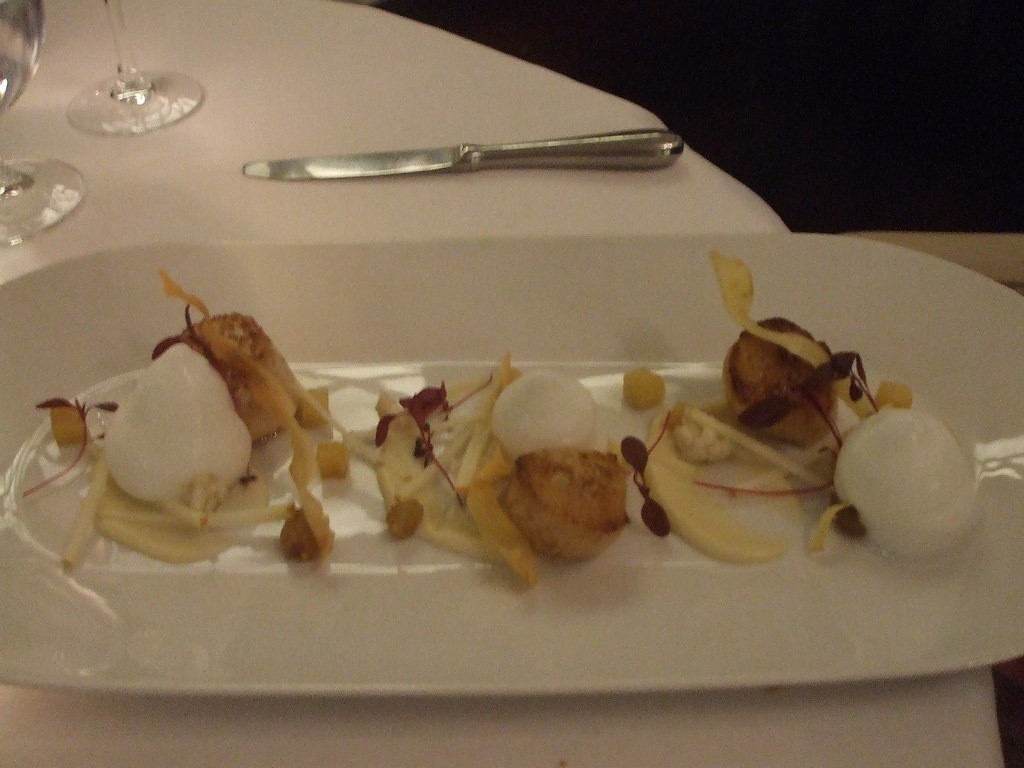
[243,128,685,181]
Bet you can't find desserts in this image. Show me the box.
[836,406,983,554]
[492,370,599,457]
[104,346,249,500]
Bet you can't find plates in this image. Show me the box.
[0,235,1024,696]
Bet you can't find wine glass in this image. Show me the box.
[0,0,87,242]
[68,0,203,134]
